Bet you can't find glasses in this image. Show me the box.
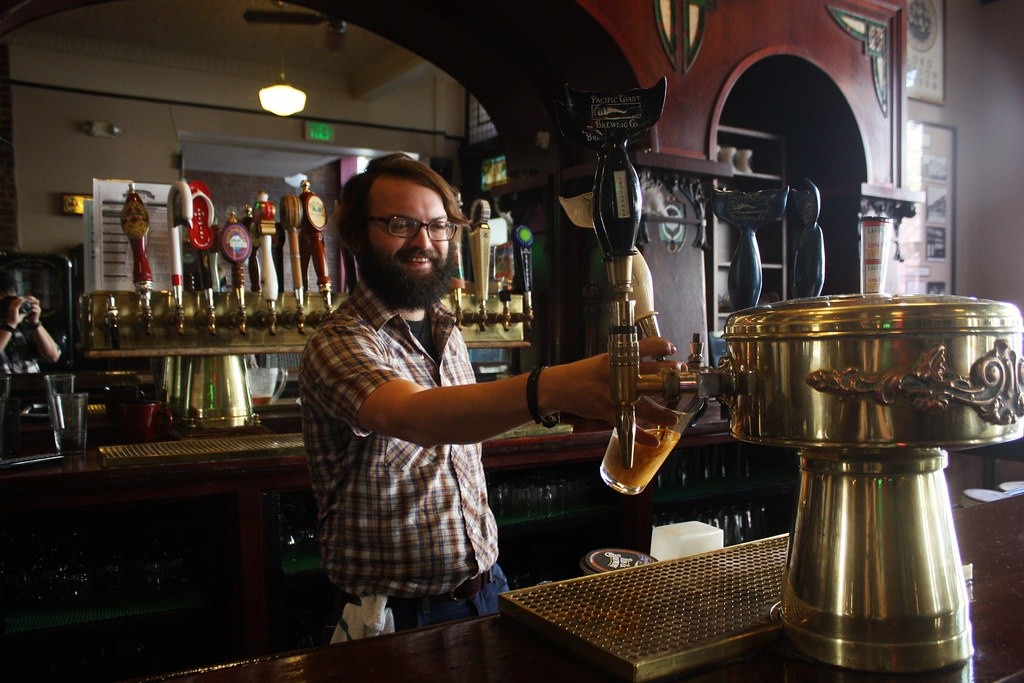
[368,215,458,242]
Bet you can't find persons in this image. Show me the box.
[298,151,690,634]
[0,272,62,463]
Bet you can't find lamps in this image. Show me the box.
[258,0,307,118]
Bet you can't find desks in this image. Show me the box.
[150,482,1024,683]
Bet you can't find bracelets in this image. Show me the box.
[2,321,17,334]
[32,321,42,330]
[524,366,561,428]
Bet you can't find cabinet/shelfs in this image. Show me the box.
[0,413,797,683]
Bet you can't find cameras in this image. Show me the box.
[19,301,34,315]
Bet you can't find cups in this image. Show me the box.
[599,384,706,494]
[44,374,75,396]
[487,477,586,519]
[48,393,88,456]
[250,367,288,405]
[119,403,173,443]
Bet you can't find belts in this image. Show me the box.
[388,566,494,608]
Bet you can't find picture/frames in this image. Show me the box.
[902,0,948,109]
[908,117,959,296]
